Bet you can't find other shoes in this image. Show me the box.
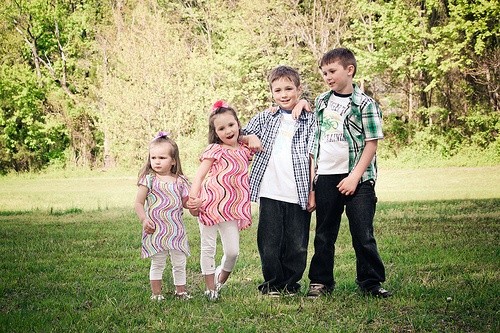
[214,265,225,293]
[268,282,301,298]
[355,283,393,299]
[174,291,192,301]
[150,295,165,303]
[307,283,327,300]
[204,290,219,303]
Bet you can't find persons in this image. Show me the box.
[291,48,388,296]
[135,131,202,302]
[189,100,256,302]
[238,66,317,297]
